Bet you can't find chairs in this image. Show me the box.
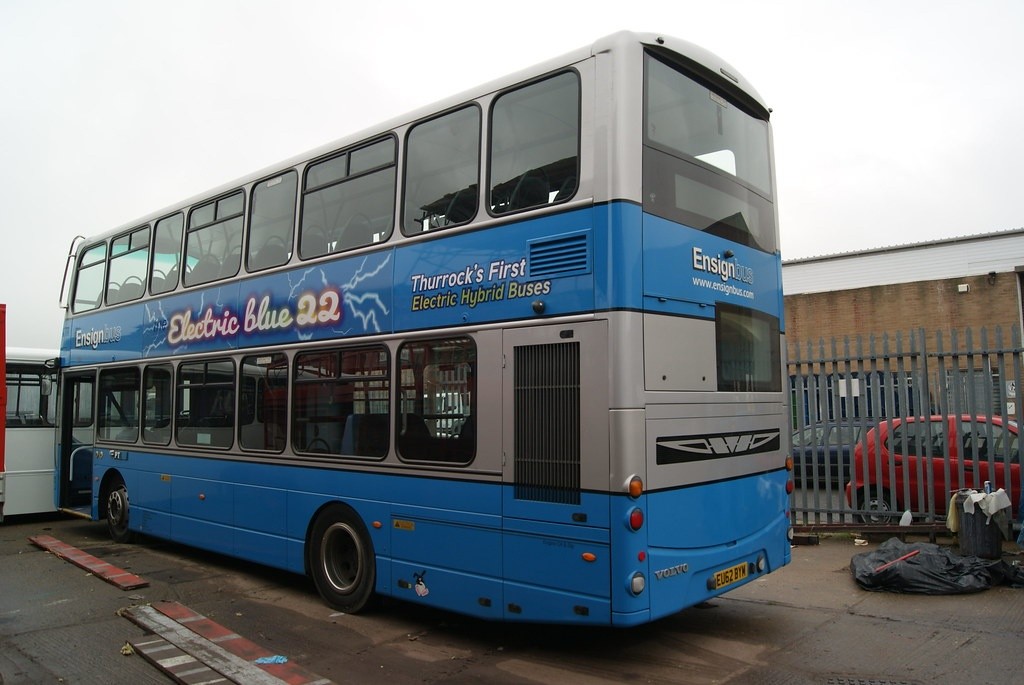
[115,412,472,462]
[96,211,372,311]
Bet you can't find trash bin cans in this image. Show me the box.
[955,488,1002,559]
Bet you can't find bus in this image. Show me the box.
[53,32,794,629]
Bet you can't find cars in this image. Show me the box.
[792,417,890,486]
[846,414,1024,536]
